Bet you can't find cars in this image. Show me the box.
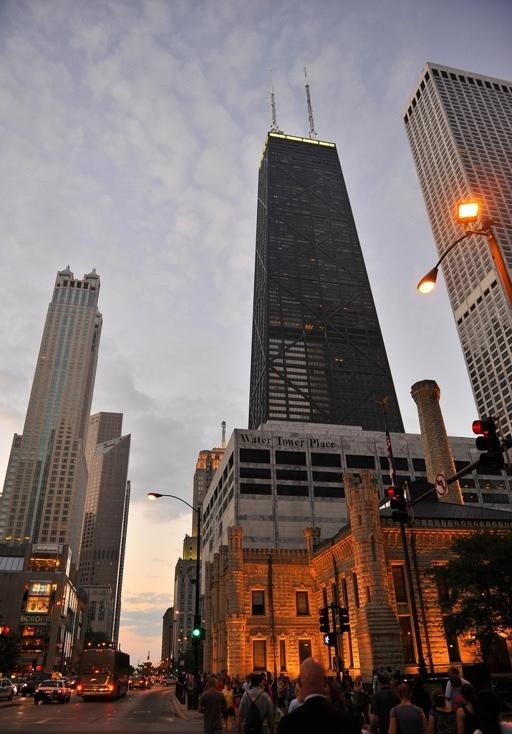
[0,671,79,704]
[128,674,178,690]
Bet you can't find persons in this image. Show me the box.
[200,657,480,734]
[177,671,194,687]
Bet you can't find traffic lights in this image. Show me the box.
[0,624,11,637]
[34,665,44,673]
[387,487,408,524]
[319,602,350,645]
[472,419,504,468]
[193,628,205,640]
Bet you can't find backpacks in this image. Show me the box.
[243,689,266,734]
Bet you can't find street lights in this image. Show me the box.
[417,202,512,309]
[372,396,427,682]
[147,493,200,672]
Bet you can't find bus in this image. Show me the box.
[77,642,129,697]
